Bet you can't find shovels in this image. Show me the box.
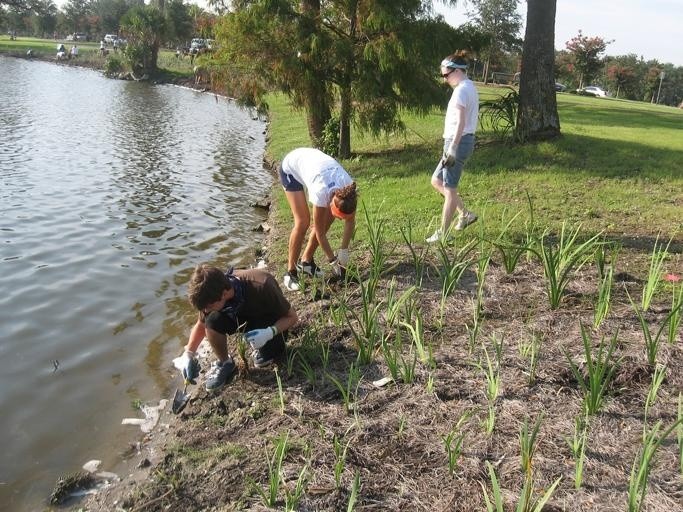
[172,377,193,414]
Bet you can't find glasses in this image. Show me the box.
[440,67,456,80]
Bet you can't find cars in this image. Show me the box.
[576,86,607,97]
[73,32,86,42]
[555,83,566,92]
[104,35,117,43]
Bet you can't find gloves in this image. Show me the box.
[335,248,349,267]
[243,326,274,351]
[180,351,201,385]
[440,143,463,169]
[327,257,342,278]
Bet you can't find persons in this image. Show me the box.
[99,40,107,57]
[277,146,358,293]
[55,44,65,59]
[113,37,117,51]
[69,45,78,59]
[422,49,479,243]
[178,264,299,392]
[25,49,33,57]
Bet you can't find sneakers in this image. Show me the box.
[282,269,302,292]
[424,230,452,244]
[252,348,275,368]
[295,256,324,278]
[204,354,237,393]
[453,210,478,232]
